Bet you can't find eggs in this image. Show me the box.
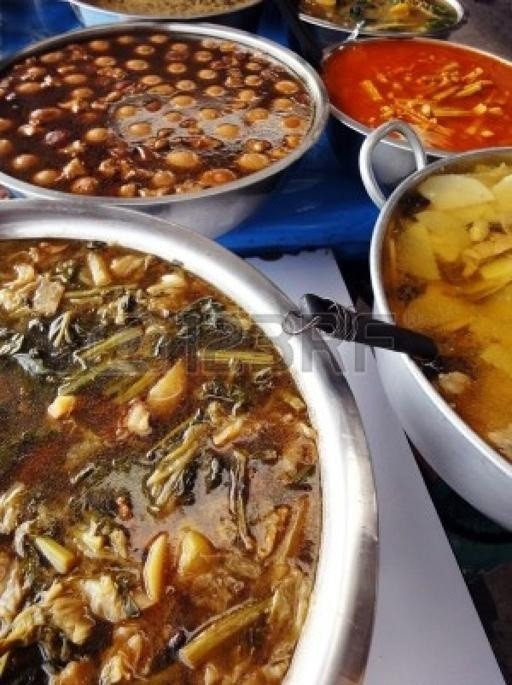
[0,35,302,195]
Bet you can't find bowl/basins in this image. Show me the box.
[1,2,512,685]
